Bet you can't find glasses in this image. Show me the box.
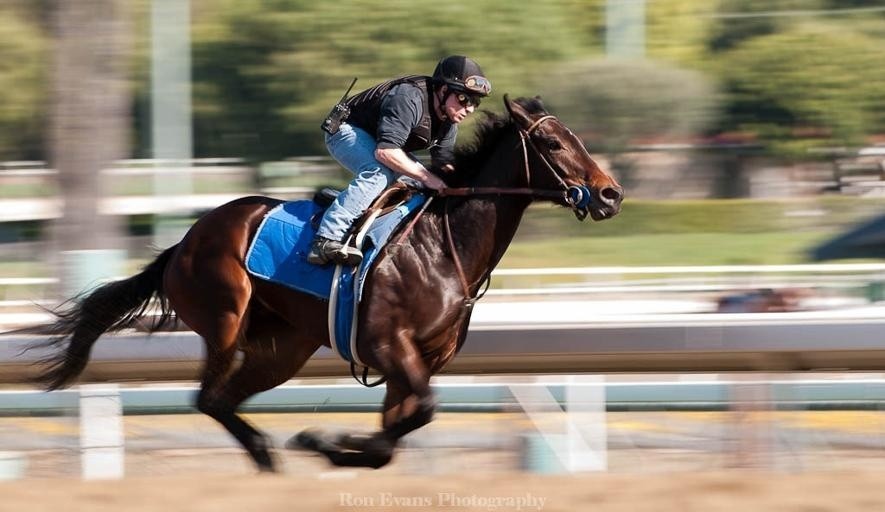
[456,93,482,109]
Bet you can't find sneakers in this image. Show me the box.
[306,239,363,266]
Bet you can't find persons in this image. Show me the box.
[306,54,495,267]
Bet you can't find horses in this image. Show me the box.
[0,92,626,475]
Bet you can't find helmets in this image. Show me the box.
[432,55,493,97]
